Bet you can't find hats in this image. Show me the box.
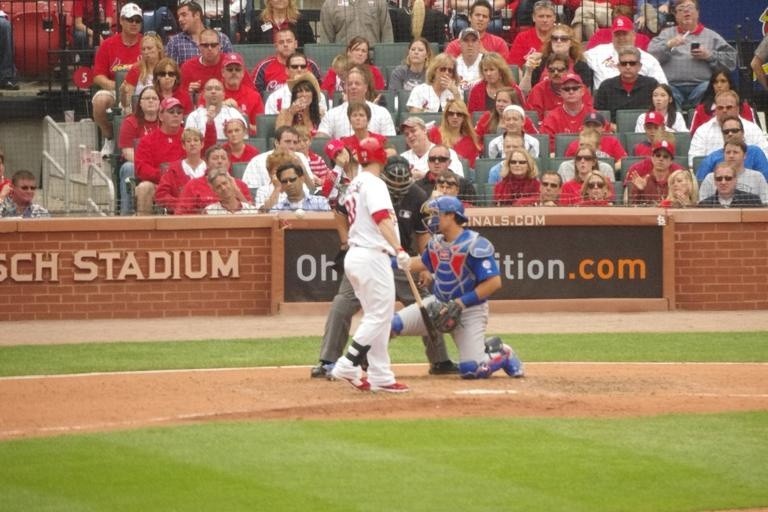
[613,17,634,32]
[645,112,665,126]
[584,114,604,127]
[559,74,582,84]
[653,141,676,157]
[287,70,321,102]
[325,139,345,161]
[221,51,244,68]
[399,117,427,128]
[121,3,143,20]
[459,27,480,41]
[160,97,182,112]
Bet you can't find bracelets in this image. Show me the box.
[340,240,349,246]
[390,255,398,268]
[423,268,429,272]
[461,290,481,307]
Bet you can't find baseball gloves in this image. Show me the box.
[431,299,462,333]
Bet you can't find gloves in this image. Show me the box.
[396,252,411,273]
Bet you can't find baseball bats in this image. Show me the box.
[398,256,443,349]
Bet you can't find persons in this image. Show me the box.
[332,133,412,392]
[0,1,768,215]
[313,154,460,378]
[323,196,523,380]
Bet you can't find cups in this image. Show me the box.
[64,111,74,123]
[501,9,512,31]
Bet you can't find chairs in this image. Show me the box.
[90,43,762,216]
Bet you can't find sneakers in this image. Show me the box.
[430,360,460,374]
[311,361,407,392]
[2,80,20,90]
[498,345,522,378]
[102,138,115,160]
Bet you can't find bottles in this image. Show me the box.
[120,81,130,107]
[99,5,105,23]
[440,24,450,44]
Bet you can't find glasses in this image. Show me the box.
[577,155,594,161]
[291,64,308,70]
[200,42,221,48]
[439,67,455,74]
[21,184,38,191]
[438,175,457,186]
[226,67,242,73]
[447,111,463,117]
[159,70,176,77]
[587,181,606,188]
[126,17,141,24]
[550,33,569,42]
[281,176,297,185]
[510,160,528,165]
[563,87,581,91]
[620,61,636,67]
[715,175,733,182]
[549,66,567,72]
[543,181,559,189]
[721,127,741,135]
[428,156,448,163]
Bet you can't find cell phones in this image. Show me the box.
[691,43,700,50]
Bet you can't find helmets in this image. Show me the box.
[420,194,467,234]
[356,137,387,164]
[384,155,414,197]
